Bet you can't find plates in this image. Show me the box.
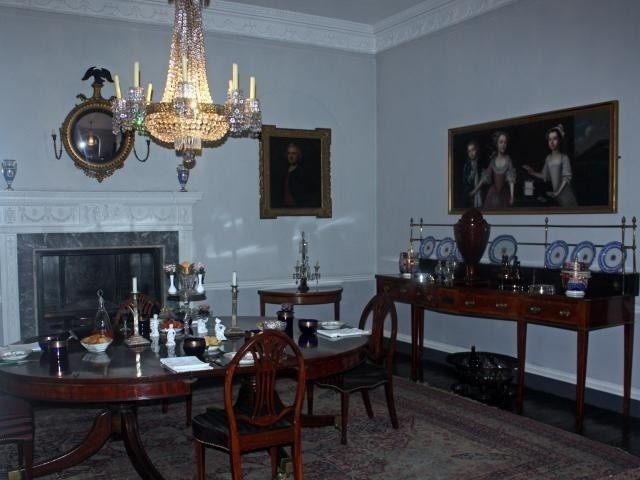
[160,328,183,333]
[598,240,627,273]
[436,237,454,260]
[545,240,569,269]
[256,320,286,331]
[0,348,33,361]
[488,234,518,263]
[318,321,345,329]
[205,345,219,352]
[454,247,464,263]
[223,351,260,365]
[161,333,182,336]
[419,236,436,259]
[571,241,596,267]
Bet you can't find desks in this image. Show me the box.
[257,286,344,322]
[373,271,635,434]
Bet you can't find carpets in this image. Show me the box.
[1,370,640,480]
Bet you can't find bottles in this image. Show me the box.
[94,289,113,350]
[496,254,521,291]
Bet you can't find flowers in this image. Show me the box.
[162,263,183,275]
[192,262,208,274]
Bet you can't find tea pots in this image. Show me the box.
[198,304,210,315]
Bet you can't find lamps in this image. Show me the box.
[293,230,321,291]
[108,2,263,164]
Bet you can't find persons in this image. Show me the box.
[467,129,518,207]
[272,141,321,208]
[522,124,586,207]
[461,140,489,208]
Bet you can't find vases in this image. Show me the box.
[196,273,205,294]
[167,274,177,295]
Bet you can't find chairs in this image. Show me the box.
[0,391,36,480]
[114,294,169,414]
[307,292,398,445]
[189,328,307,480]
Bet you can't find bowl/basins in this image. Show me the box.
[276,311,295,323]
[47,339,68,360]
[40,351,59,368]
[298,333,318,348]
[183,338,205,356]
[80,336,112,352]
[298,319,318,334]
[284,324,294,339]
[49,359,72,376]
[82,352,111,364]
[39,335,59,352]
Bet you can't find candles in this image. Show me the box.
[231,270,237,287]
[132,275,137,293]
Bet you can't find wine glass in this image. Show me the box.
[120,311,131,331]
[2,159,17,191]
[176,164,189,191]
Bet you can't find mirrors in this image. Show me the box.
[50,66,150,183]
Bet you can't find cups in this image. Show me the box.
[138,316,150,340]
[281,303,294,311]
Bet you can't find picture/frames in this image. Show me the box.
[447,99,619,217]
[258,124,333,219]
